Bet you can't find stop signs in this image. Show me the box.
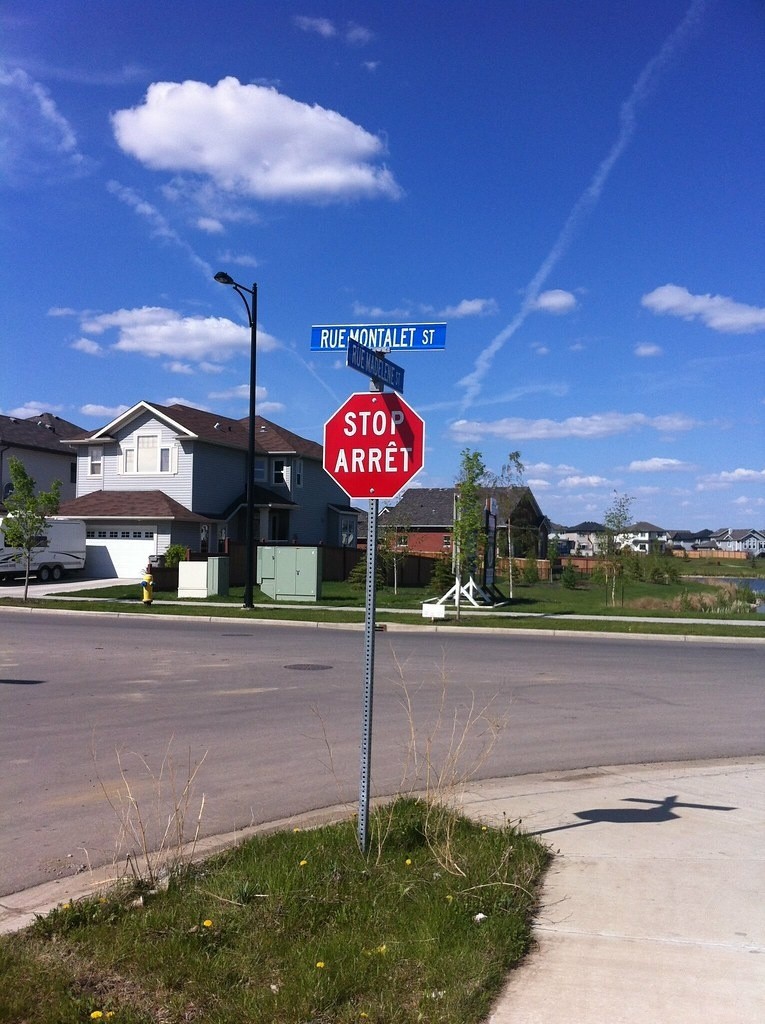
[325,391,426,500]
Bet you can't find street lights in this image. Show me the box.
[213,271,257,612]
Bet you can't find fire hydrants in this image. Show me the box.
[140,573,155,606]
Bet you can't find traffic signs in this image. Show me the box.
[346,336,405,395]
[308,322,447,353]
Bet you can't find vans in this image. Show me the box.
[0,515,87,587]
